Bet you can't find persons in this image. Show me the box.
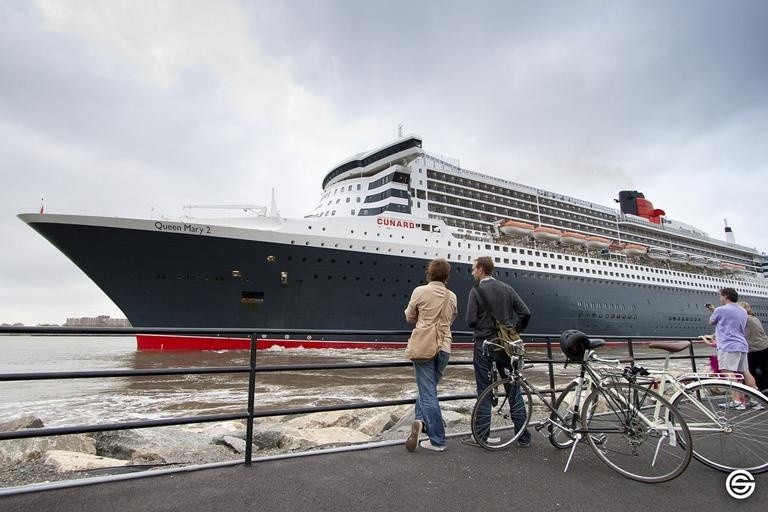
[703,288,765,411]
[737,301,768,396]
[402,258,458,452]
[697,333,717,348]
[465,257,533,447]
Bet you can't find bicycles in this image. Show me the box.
[548,340,767,475]
[470,336,693,484]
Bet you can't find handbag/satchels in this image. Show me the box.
[403,325,444,362]
[496,319,525,359]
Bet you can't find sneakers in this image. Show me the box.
[717,400,758,411]
[420,438,446,451]
[517,441,530,447]
[405,420,423,452]
[461,437,487,448]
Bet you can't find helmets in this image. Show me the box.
[559,328,590,360]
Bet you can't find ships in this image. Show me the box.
[17,122,767,356]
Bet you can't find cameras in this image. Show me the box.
[703,303,711,308]
